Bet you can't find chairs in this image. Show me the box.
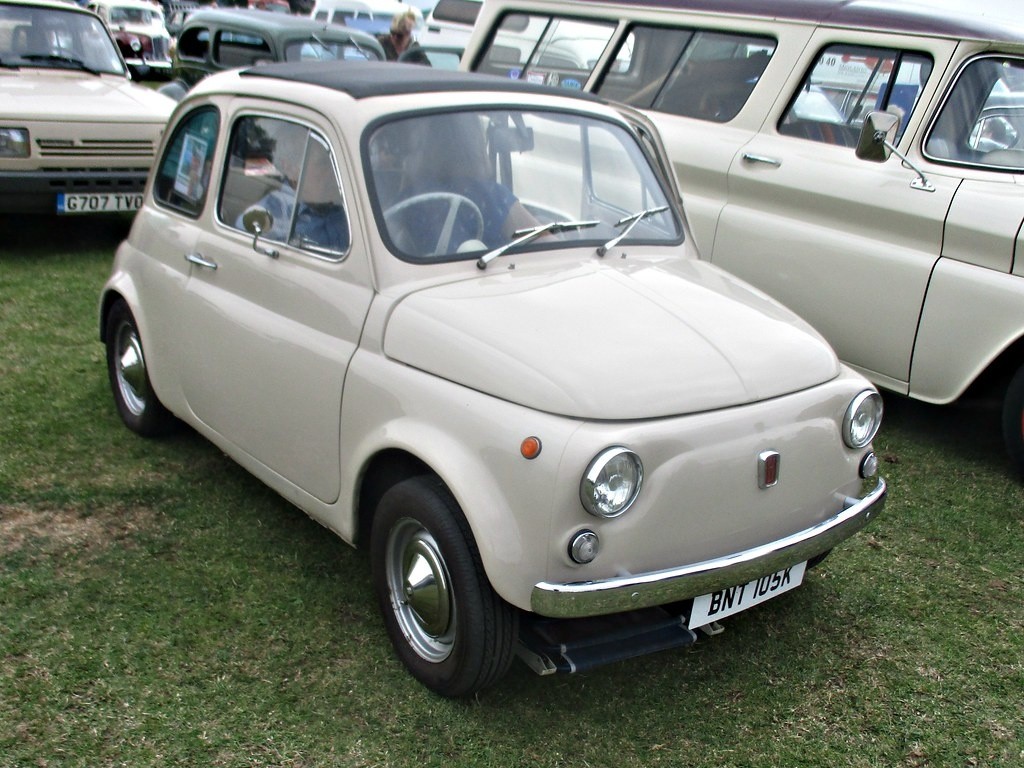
[799,118,861,148]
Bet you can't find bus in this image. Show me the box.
[420,4,1024,478]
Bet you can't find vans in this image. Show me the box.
[305,0,436,60]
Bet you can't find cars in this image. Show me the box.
[165,7,389,118]
[82,0,167,63]
[93,58,887,699]
[394,2,1021,167]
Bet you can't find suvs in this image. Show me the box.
[0,0,174,231]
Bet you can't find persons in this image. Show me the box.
[376,11,433,68]
[398,122,559,258]
[212,0,274,12]
[234,125,348,254]
